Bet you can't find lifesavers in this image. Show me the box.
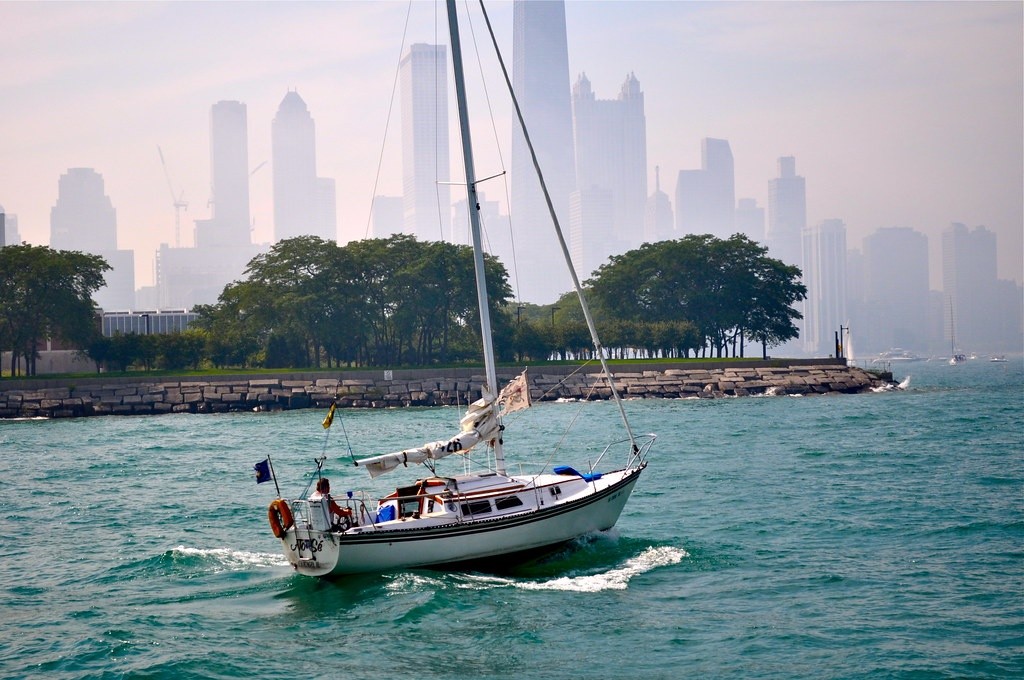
[268,499,292,538]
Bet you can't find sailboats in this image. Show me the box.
[267,0,652,578]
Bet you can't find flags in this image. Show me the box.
[322,402,336,428]
[252,459,272,485]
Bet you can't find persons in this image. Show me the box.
[309,478,352,532]
